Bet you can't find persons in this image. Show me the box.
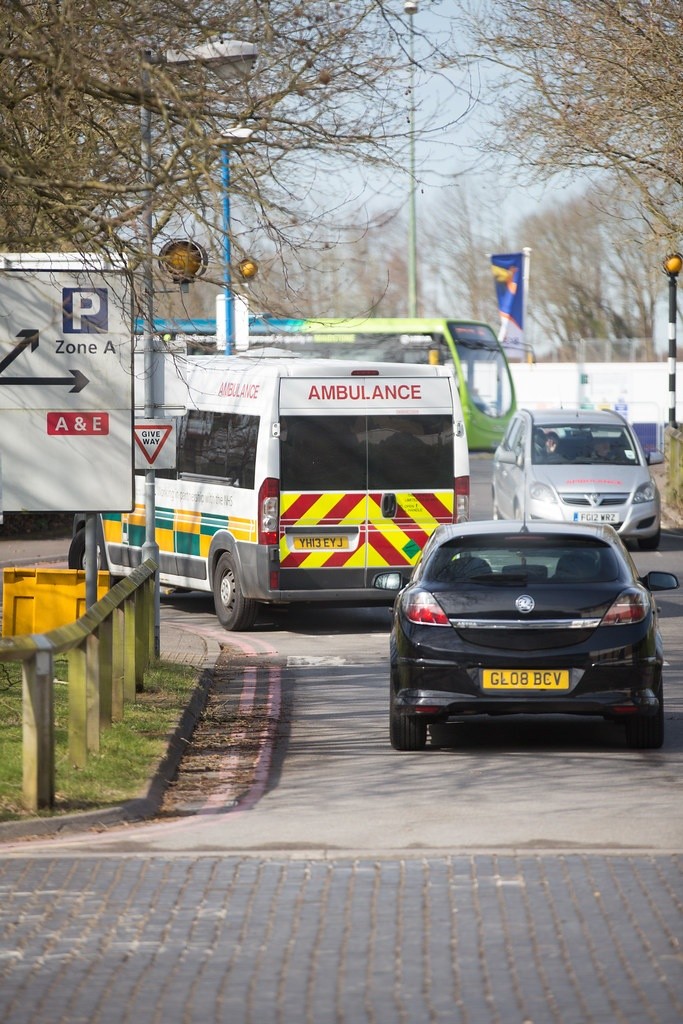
[546,555,586,585]
[541,431,560,464]
[594,438,614,462]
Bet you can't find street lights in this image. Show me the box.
[215,123,251,354]
[405,1,418,320]
[143,40,261,658]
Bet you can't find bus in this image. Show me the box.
[452,362,683,456]
[133,318,539,454]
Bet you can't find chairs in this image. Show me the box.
[552,553,597,580]
[448,557,491,577]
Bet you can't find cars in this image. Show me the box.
[371,515,679,755]
[494,411,664,548]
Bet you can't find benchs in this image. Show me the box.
[492,564,551,583]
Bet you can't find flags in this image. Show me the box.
[490,252,523,332]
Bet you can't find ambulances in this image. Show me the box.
[69,344,471,633]
